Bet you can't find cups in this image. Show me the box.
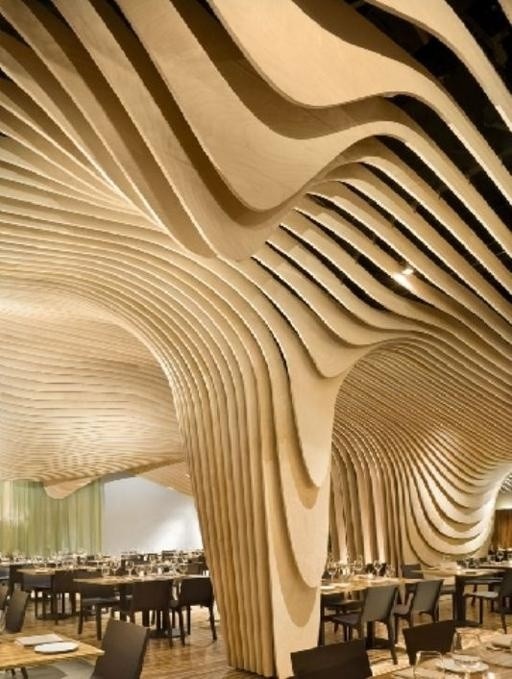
[413,650,444,679]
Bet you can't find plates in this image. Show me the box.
[437,659,487,673]
[34,641,79,655]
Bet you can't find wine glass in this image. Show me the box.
[496,550,504,565]
[463,555,471,570]
[79,554,187,581]
[327,559,396,587]
[450,633,483,677]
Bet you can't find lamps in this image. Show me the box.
[400,263,415,277]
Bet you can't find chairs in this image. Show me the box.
[5,590,28,632]
[402,621,454,664]
[76,584,118,641]
[121,579,172,648]
[394,579,443,643]
[333,584,398,664]
[172,578,218,647]
[1,548,512,625]
[85,620,151,678]
[289,638,374,678]
